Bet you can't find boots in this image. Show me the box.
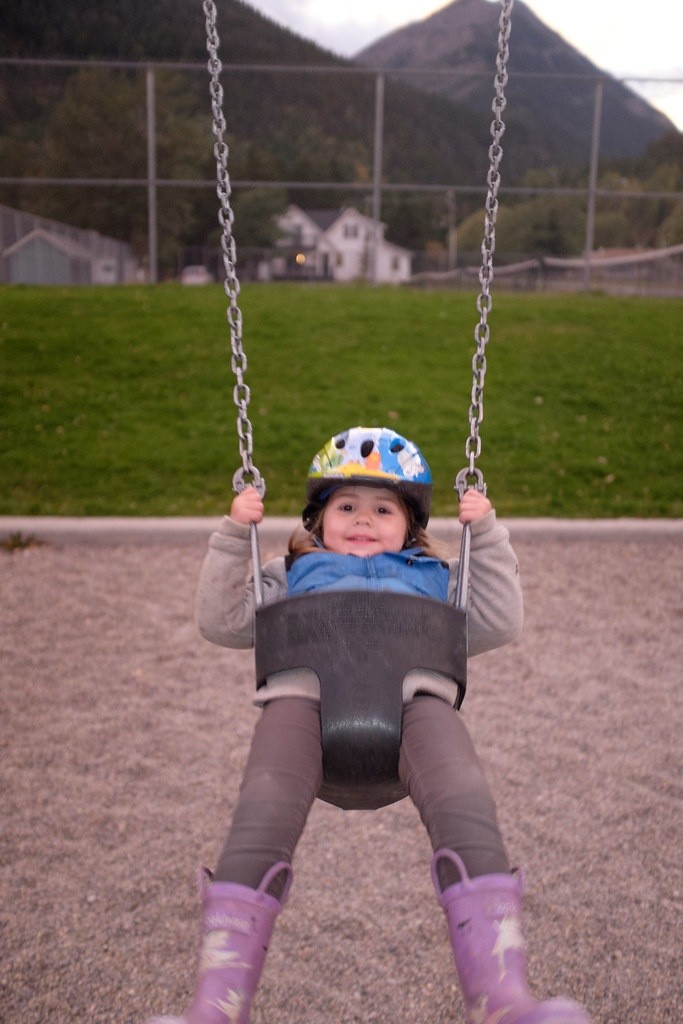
[430,848,593,1024]
[144,861,293,1024]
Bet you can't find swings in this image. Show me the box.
[202,2,520,814]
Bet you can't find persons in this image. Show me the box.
[145,426,590,1024]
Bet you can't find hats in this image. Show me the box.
[301,426,433,536]
